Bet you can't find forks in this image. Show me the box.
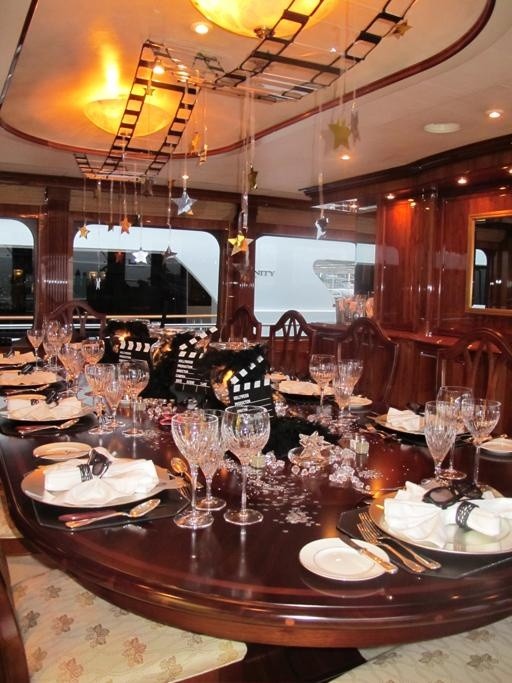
[355,512,442,571]
[356,521,426,574]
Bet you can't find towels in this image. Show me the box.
[386,405,425,432]
[383,480,512,550]
[0,348,36,365]
[1,364,57,385]
[43,446,159,506]
[7,391,82,419]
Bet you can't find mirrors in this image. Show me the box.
[465,209,512,316]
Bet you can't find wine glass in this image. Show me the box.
[461,398,501,489]
[42,320,72,372]
[170,412,219,528]
[221,404,271,526]
[85,363,116,436]
[334,359,352,421]
[187,408,230,511]
[346,358,363,421]
[119,359,149,437]
[420,401,459,488]
[55,338,83,396]
[24,325,46,371]
[436,385,474,480]
[104,365,128,428]
[81,340,106,366]
[309,353,334,425]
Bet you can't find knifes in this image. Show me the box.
[338,534,399,576]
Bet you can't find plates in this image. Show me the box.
[481,437,512,456]
[0,401,92,421]
[32,442,92,461]
[1,353,29,364]
[298,537,390,583]
[350,396,372,409]
[369,484,512,556]
[343,395,373,410]
[20,457,169,509]
[376,413,469,435]
[0,369,54,387]
[273,380,335,397]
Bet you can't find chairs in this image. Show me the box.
[333,318,399,406]
[269,310,318,380]
[43,302,105,340]
[324,616,512,683]
[435,328,511,440]
[1,542,248,683]
[222,304,262,345]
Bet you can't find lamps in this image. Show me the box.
[83,99,173,138]
[191,0,339,40]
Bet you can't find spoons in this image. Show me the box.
[54,497,163,529]
[17,418,80,435]
